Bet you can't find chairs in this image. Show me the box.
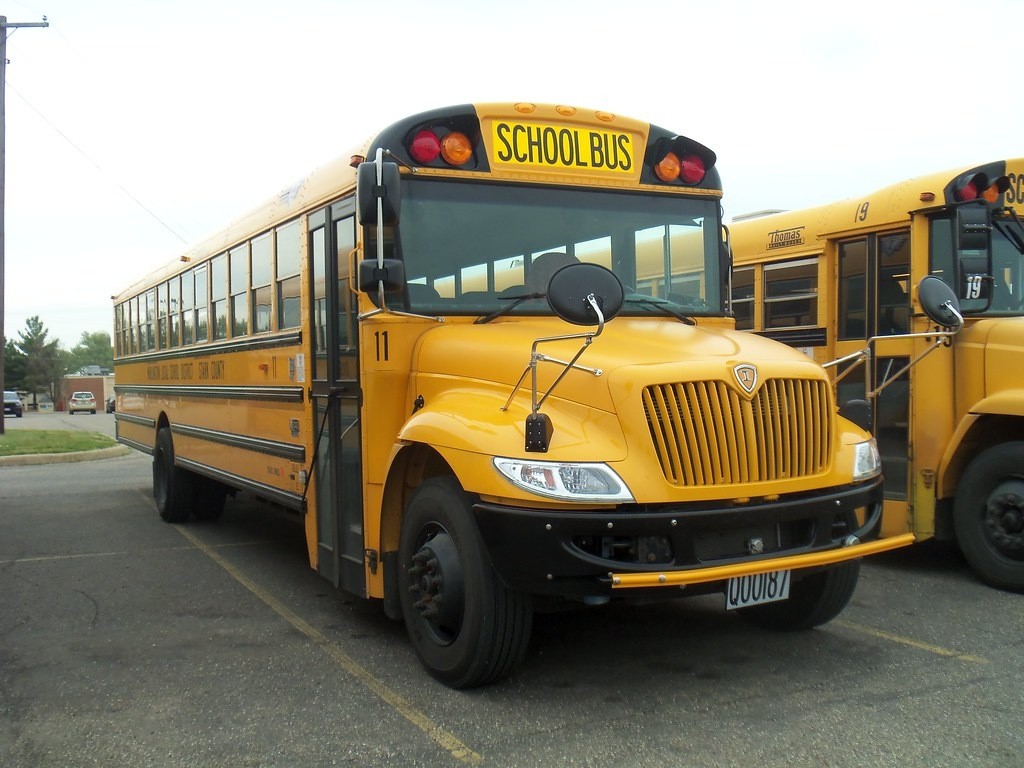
[408,284,441,304]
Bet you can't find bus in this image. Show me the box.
[433,155,1024,598]
[107,101,918,693]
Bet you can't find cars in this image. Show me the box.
[3,391,22,418]
[104,395,115,414]
[69,392,96,415]
